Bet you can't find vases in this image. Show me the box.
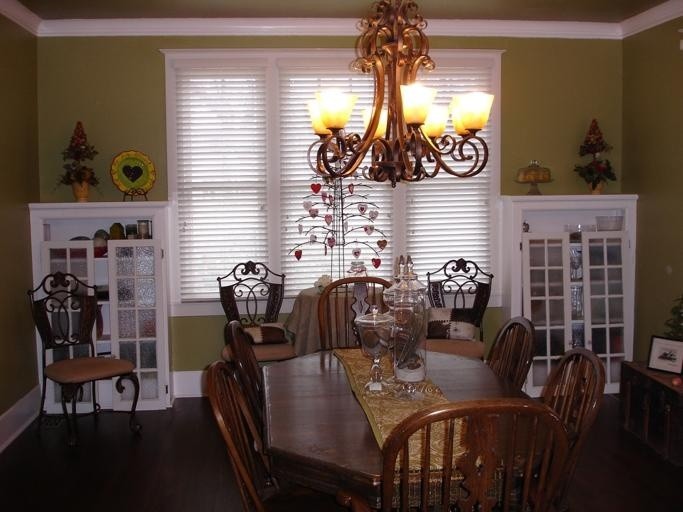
[587,178,608,195]
[69,181,91,202]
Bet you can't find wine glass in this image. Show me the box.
[354,305,393,393]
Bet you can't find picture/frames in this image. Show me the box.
[647,334,683,376]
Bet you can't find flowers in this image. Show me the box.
[572,117,617,188]
[48,120,108,197]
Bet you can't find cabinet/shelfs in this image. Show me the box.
[621,360,683,470]
[488,194,639,397]
[27,201,175,416]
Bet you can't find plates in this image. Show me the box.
[110,150,156,196]
[564,224,596,233]
[596,215,624,231]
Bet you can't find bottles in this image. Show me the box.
[573,324,584,347]
[571,287,582,320]
[570,247,582,281]
[393,255,427,383]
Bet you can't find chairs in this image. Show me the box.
[26,271,142,446]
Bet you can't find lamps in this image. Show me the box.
[307,0,496,188]
